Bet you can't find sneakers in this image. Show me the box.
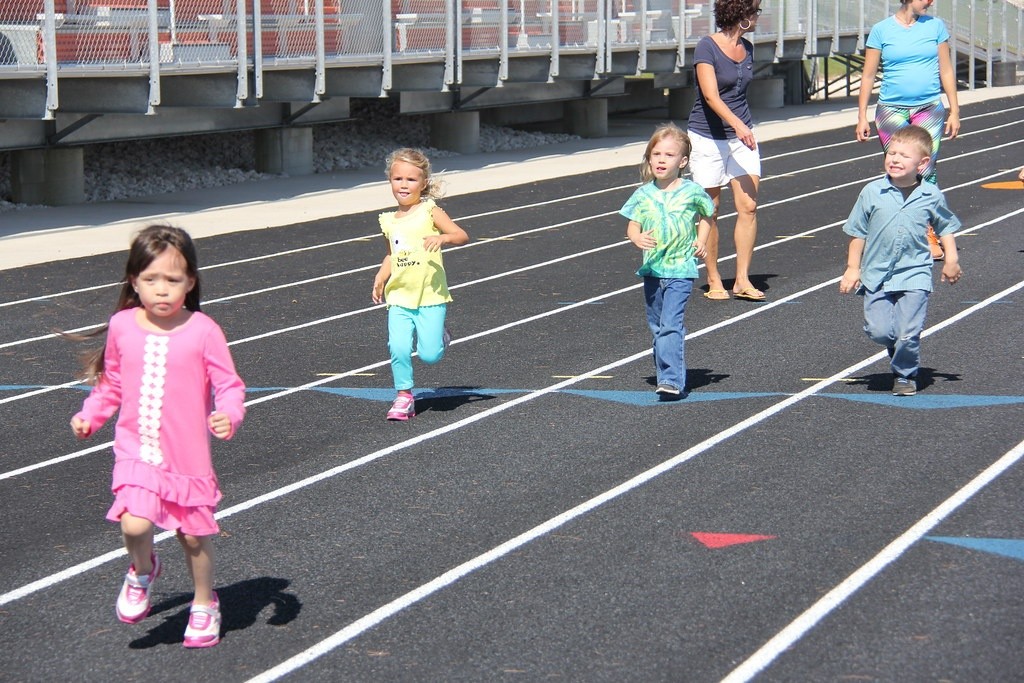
[928,226,944,260]
[182,590,222,649]
[887,347,895,358]
[655,384,680,397]
[443,322,451,349]
[386,393,416,420]
[892,377,917,396]
[115,547,161,624]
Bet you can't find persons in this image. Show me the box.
[618,122,715,397]
[837,124,963,396]
[68,224,248,649]
[854,0,962,259]
[685,0,766,302]
[370,148,469,420]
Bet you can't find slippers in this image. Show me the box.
[703,290,730,300]
[732,288,766,300]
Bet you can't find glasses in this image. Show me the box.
[756,8,763,16]
